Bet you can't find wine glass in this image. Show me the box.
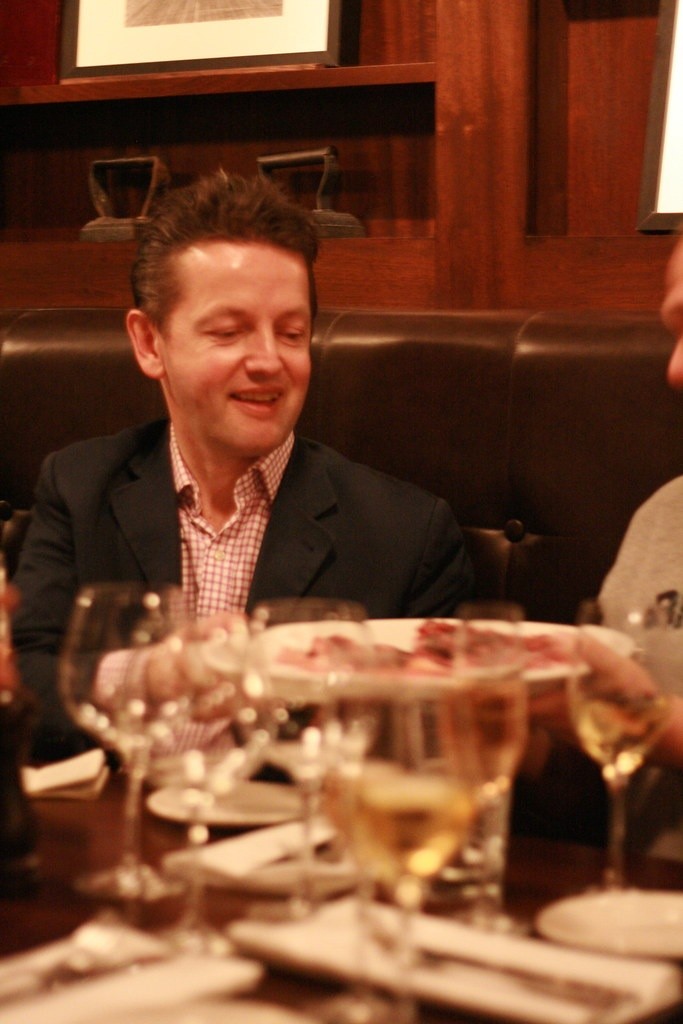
[60,581,683,1024]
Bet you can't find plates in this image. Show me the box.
[161,850,367,896]
[534,887,683,963]
[1,884,683,1024]
[207,615,638,700]
[146,774,321,826]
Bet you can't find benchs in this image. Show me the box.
[0,301,683,628]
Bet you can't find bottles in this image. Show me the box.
[0,564,35,902]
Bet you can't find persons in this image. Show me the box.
[14,166,683,865]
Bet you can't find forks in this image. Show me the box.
[361,915,627,1010]
